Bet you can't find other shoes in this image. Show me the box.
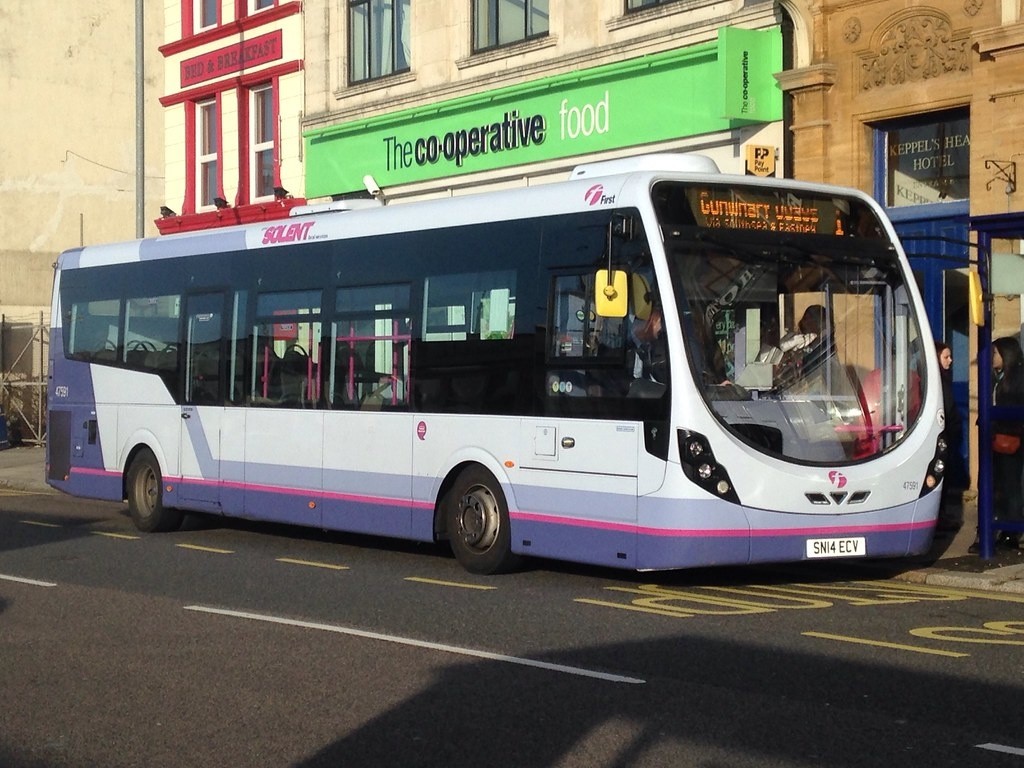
[968,526,982,554]
[993,530,1019,549]
[937,518,960,532]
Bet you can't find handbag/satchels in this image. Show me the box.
[990,431,1021,454]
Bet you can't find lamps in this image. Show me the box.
[213,198,229,207]
[273,187,288,198]
[160,206,174,216]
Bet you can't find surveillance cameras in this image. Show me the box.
[364,175,380,196]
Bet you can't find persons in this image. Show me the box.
[553,304,955,460]
[968,338,1024,554]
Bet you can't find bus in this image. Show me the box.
[42,155,996,577]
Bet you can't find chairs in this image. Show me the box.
[92,340,366,403]
